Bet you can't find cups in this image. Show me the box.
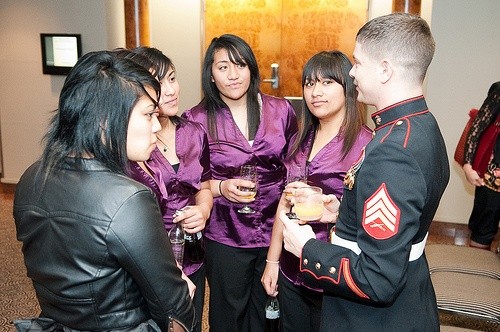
[293,187,322,220]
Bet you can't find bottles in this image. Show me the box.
[264,296,280,332]
[168,211,185,266]
[184,207,206,264]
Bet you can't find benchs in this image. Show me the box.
[424,244,500,332]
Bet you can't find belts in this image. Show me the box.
[328,225,429,262]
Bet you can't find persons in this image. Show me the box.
[261,13,450,332]
[182,35,297,332]
[462,82,499,253]
[10,46,214,332]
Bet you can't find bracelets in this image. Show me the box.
[265,259,280,265]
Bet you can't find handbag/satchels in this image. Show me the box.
[454,110,500,178]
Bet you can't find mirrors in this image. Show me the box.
[40,32,83,76]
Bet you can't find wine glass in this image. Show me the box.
[286,165,308,219]
[237,165,257,213]
